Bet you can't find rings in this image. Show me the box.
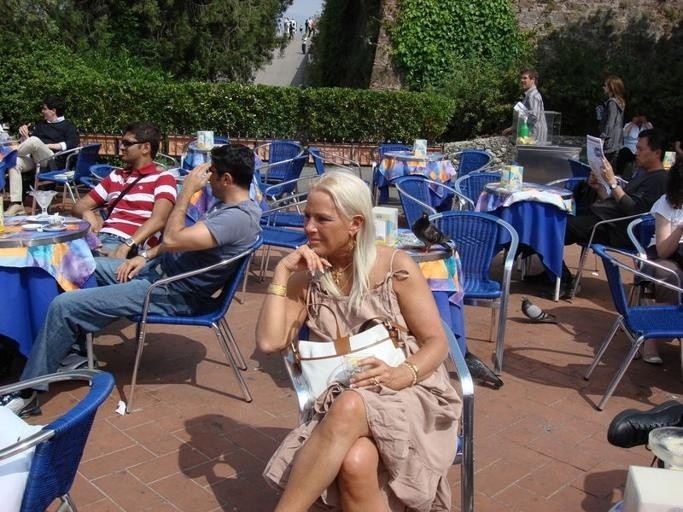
[371,376,379,385]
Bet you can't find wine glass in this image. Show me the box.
[26,191,59,215]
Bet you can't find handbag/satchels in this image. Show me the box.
[286,302,407,411]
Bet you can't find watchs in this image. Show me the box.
[609,182,619,189]
[124,238,134,247]
[137,249,149,261]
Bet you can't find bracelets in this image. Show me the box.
[266,282,288,297]
[398,361,419,387]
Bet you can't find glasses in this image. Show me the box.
[122,139,145,146]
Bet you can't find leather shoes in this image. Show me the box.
[639,345,663,364]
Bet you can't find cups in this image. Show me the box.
[48,215,65,228]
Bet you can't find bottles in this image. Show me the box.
[520,119,529,138]
[597,104,603,120]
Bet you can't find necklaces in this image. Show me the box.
[332,262,352,284]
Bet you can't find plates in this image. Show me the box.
[43,224,67,231]
[23,225,42,230]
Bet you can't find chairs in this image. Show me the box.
[619,214,683,333]
[256,200,308,283]
[448,148,495,198]
[454,170,502,211]
[253,140,305,185]
[260,173,322,282]
[308,145,372,189]
[394,174,477,232]
[0,367,116,512]
[410,210,520,378]
[254,153,310,215]
[30,141,103,216]
[80,176,101,189]
[521,176,601,284]
[370,143,413,205]
[151,152,177,170]
[569,210,650,304]
[297,317,475,511]
[124,232,266,414]
[89,163,117,180]
[0,142,18,193]
[584,243,683,411]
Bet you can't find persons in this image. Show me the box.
[639,117,654,131]
[3,96,80,216]
[254,167,463,512]
[1,142,262,419]
[640,155,683,364]
[501,68,548,142]
[595,76,625,170]
[622,115,642,152]
[523,129,668,299]
[71,121,178,258]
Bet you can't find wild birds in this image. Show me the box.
[521,296,556,324]
[465,346,503,387]
[412,212,444,254]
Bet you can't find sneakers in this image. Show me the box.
[3,202,25,216]
[555,278,575,297]
[523,270,572,284]
[0,392,40,418]
